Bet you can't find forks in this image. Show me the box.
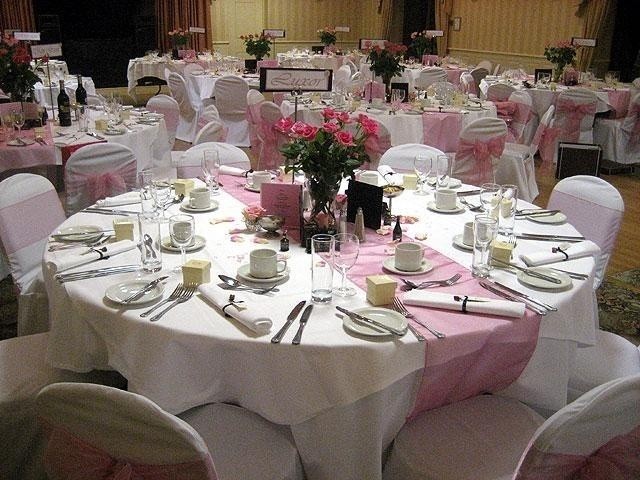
[151,281,200,322]
[416,272,465,290]
[139,282,187,318]
[390,295,445,341]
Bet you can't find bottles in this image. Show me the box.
[393,215,402,241]
[75,75,87,113]
[424,92,427,98]
[58,80,71,126]
[354,207,364,242]
[280,232,289,250]
[339,210,346,240]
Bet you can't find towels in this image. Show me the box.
[557,81,576,97]
[56,131,84,147]
[48,239,136,273]
[94,102,133,112]
[201,282,273,333]
[424,104,468,116]
[97,192,142,207]
[401,284,528,323]
[378,163,403,185]
[522,241,580,265]
[483,72,504,83]
[218,162,266,178]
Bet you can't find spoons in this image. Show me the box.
[218,275,278,292]
[144,233,158,259]
[162,195,185,207]
[462,199,482,210]
[400,277,447,289]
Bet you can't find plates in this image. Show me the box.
[105,280,166,305]
[383,255,437,274]
[245,182,261,193]
[53,225,103,242]
[527,209,566,224]
[426,201,466,214]
[517,267,573,288]
[103,128,125,137]
[137,113,162,122]
[8,139,36,147]
[163,232,207,254]
[342,307,409,336]
[451,232,474,251]
[235,263,290,283]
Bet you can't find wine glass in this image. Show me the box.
[536,73,550,90]
[472,214,496,276]
[586,66,596,83]
[333,234,360,296]
[171,215,195,277]
[414,158,432,192]
[150,183,170,225]
[1,117,14,144]
[12,112,26,144]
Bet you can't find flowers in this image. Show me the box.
[541,37,583,69]
[0,32,49,102]
[273,108,378,226]
[317,26,337,46]
[411,31,433,56]
[240,32,274,60]
[366,41,407,85]
[168,29,193,49]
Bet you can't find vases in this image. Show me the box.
[305,225,340,252]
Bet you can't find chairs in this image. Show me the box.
[460,72,477,98]
[495,105,554,204]
[445,118,507,187]
[65,142,138,218]
[0,173,66,294]
[0,331,107,480]
[193,121,228,146]
[487,84,515,103]
[384,372,640,480]
[246,89,266,157]
[540,87,596,162]
[568,328,640,401]
[498,90,532,142]
[594,93,640,175]
[178,142,251,179]
[35,382,305,479]
[419,68,449,91]
[363,119,390,170]
[146,94,180,150]
[169,73,202,144]
[546,175,624,289]
[197,105,219,136]
[259,102,286,170]
[215,75,251,146]
[379,143,449,178]
[471,67,489,86]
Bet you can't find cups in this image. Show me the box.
[279,165,294,184]
[302,183,314,211]
[100,92,123,123]
[310,234,335,304]
[392,244,427,271]
[605,71,621,90]
[169,222,194,249]
[258,213,287,240]
[363,174,379,186]
[498,184,519,236]
[249,251,287,279]
[429,80,456,109]
[463,223,477,248]
[138,214,162,271]
[388,88,406,112]
[76,109,90,135]
[434,189,456,209]
[437,156,450,188]
[246,172,273,191]
[141,188,158,227]
[481,185,498,214]
[190,189,211,210]
[148,48,172,69]
[201,151,220,192]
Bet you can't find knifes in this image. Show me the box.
[336,304,405,336]
[495,282,567,312]
[54,263,142,284]
[493,255,561,284]
[272,300,307,344]
[513,210,564,218]
[480,275,560,316]
[118,276,171,303]
[516,232,587,243]
[292,305,314,345]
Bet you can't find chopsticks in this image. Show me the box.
[79,206,140,216]
